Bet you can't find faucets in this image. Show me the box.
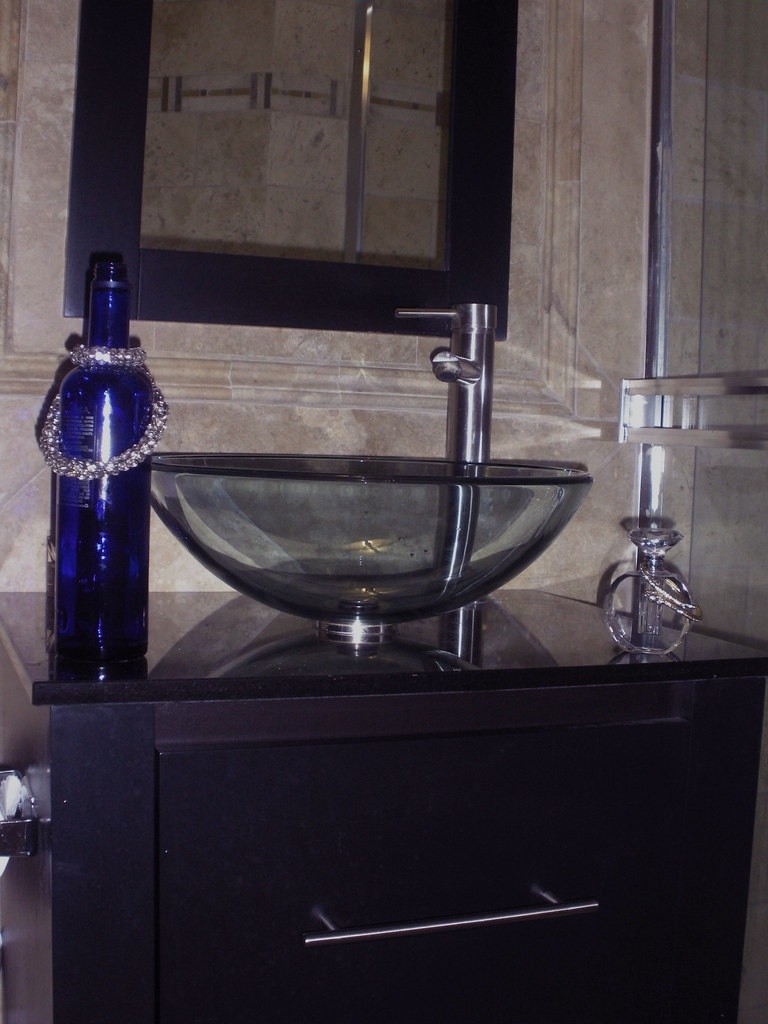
[391,295,500,473]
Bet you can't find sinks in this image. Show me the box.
[151,449,593,627]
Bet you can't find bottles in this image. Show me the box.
[54,260,155,666]
